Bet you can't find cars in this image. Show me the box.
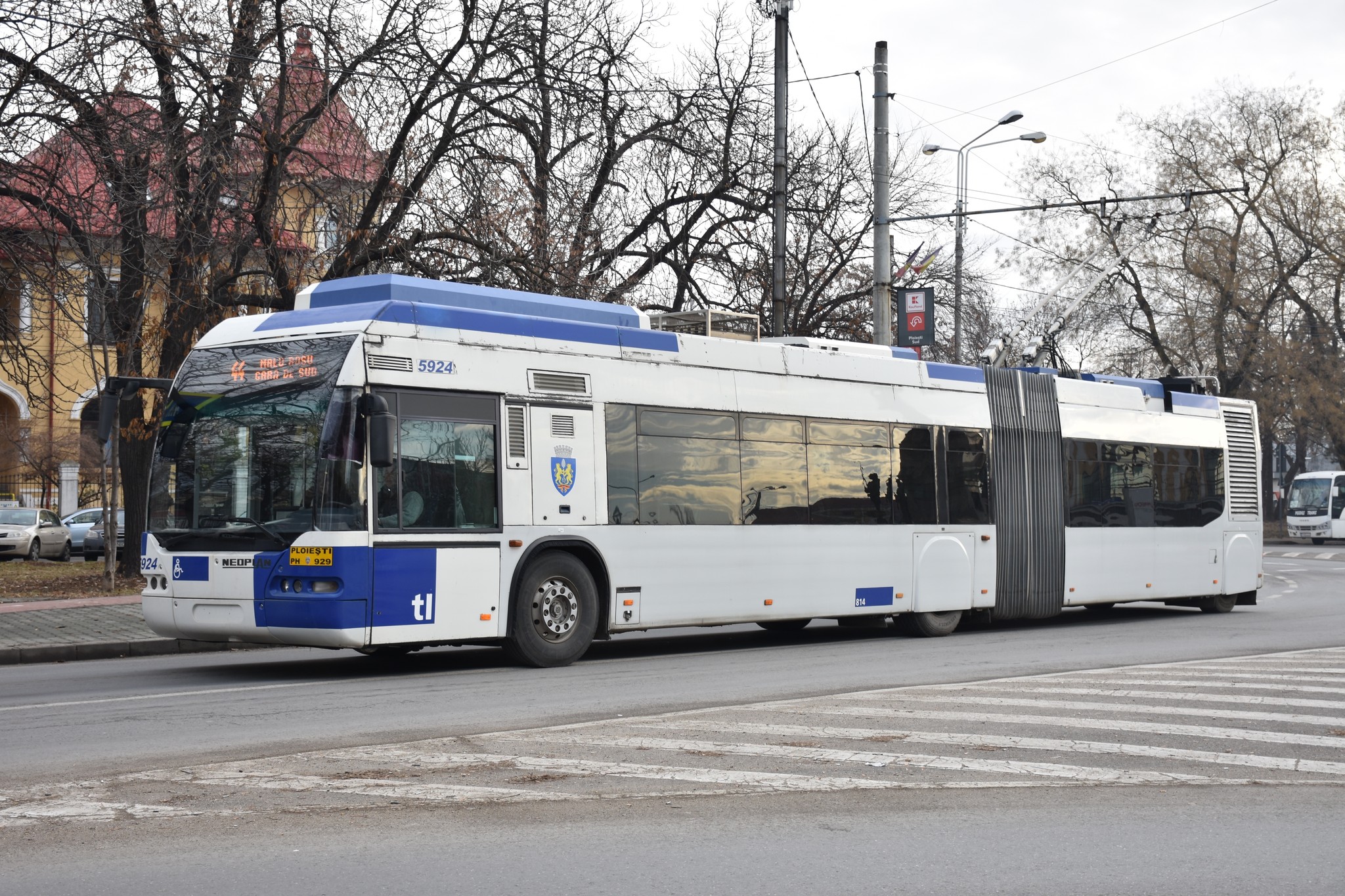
[0,507,73,563]
[83,509,126,559]
[59,508,110,556]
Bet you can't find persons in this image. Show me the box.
[1319,489,1330,505]
[310,467,352,508]
[353,459,427,528]
[1333,484,1345,508]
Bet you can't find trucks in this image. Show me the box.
[1285,471,1345,543]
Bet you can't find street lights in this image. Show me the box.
[924,111,1046,364]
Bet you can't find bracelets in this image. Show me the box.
[378,517,381,526]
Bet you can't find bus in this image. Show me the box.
[97,275,1265,674]
